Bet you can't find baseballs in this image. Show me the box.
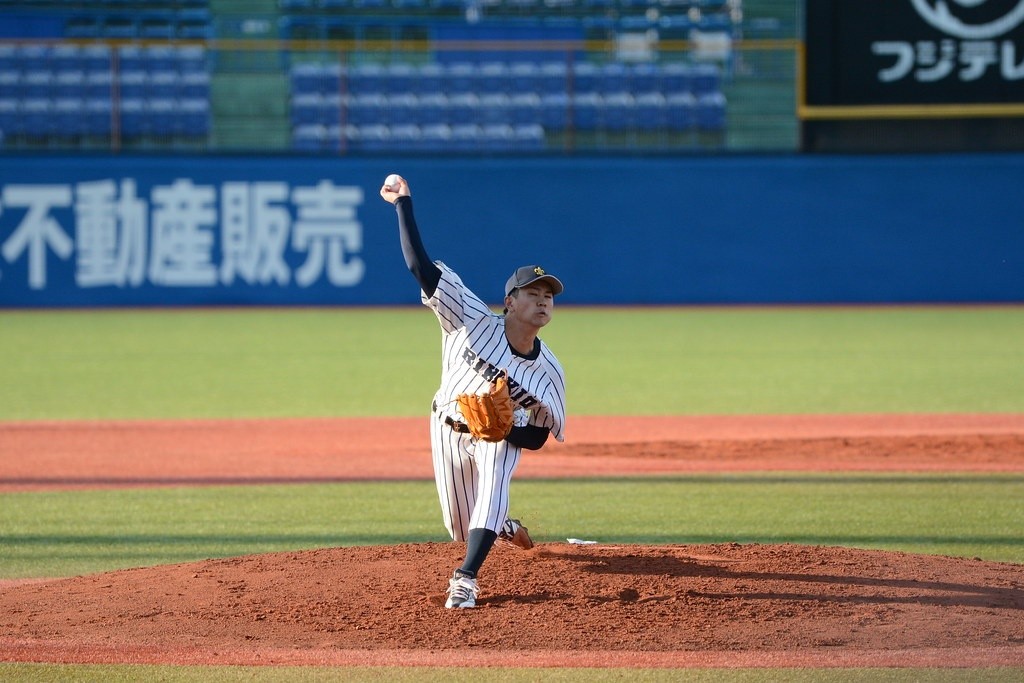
[384,174,404,193]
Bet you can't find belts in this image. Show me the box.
[432,401,470,433]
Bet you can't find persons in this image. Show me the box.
[378,175,568,609]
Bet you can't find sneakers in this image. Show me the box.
[498,516,533,550]
[445,569,480,608]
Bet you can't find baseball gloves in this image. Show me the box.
[441,367,515,444]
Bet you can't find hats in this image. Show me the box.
[505,265,563,296]
